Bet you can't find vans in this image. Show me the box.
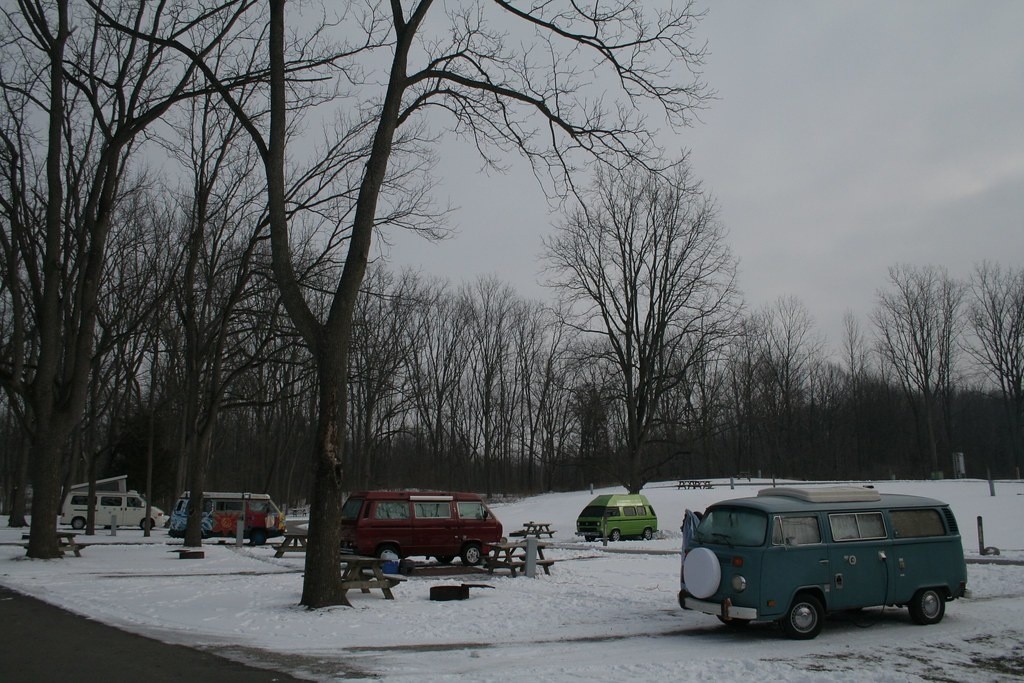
[678,482,967,640]
[338,487,504,570]
[167,489,288,547]
[58,489,166,530]
[573,493,659,542]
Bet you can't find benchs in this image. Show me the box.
[271,544,286,558]
[676,484,716,489]
[21,542,91,557]
[476,556,555,578]
[519,530,558,539]
[301,572,407,600]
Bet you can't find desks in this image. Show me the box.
[524,523,553,540]
[677,480,712,489]
[486,542,550,579]
[339,554,395,599]
[275,530,309,558]
[22,533,85,558]
